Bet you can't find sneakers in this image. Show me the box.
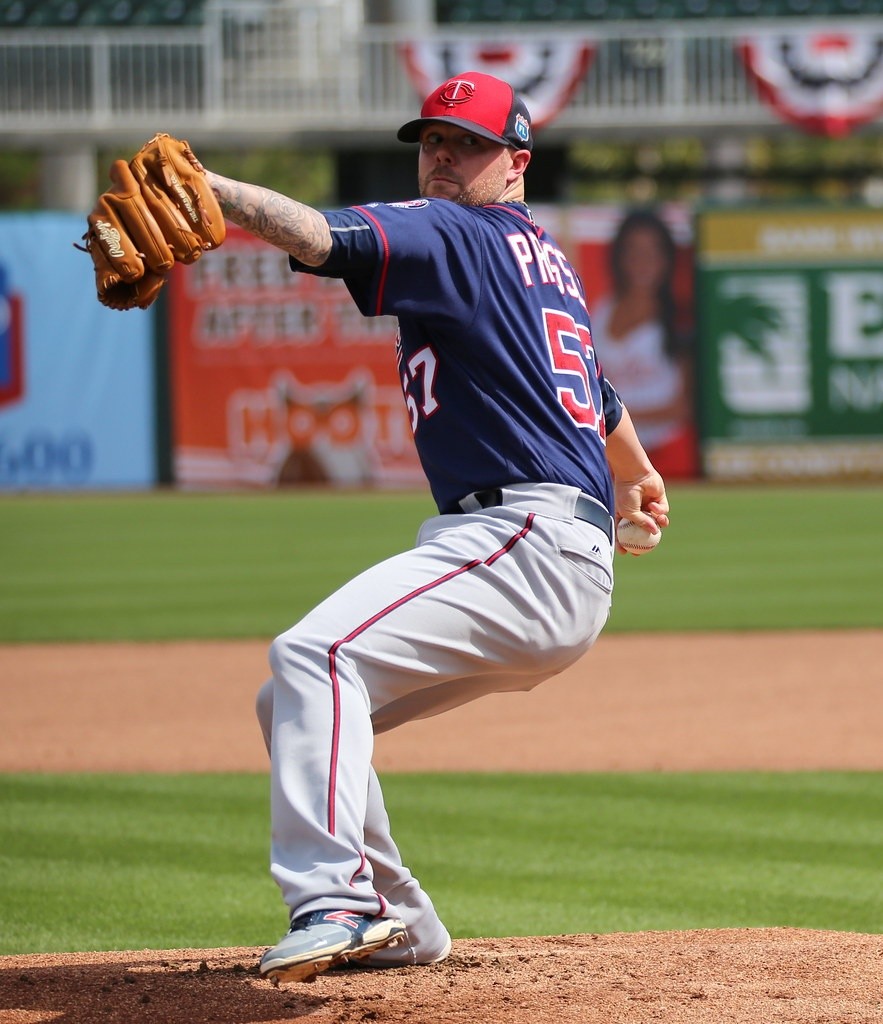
[259,910,406,990]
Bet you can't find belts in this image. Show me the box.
[445,487,612,546]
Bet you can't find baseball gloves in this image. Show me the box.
[81,131,228,313]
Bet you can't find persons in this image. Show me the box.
[71,69,672,982]
[588,207,697,480]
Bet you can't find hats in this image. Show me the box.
[396,71,534,154]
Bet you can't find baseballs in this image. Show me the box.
[616,512,663,557]
